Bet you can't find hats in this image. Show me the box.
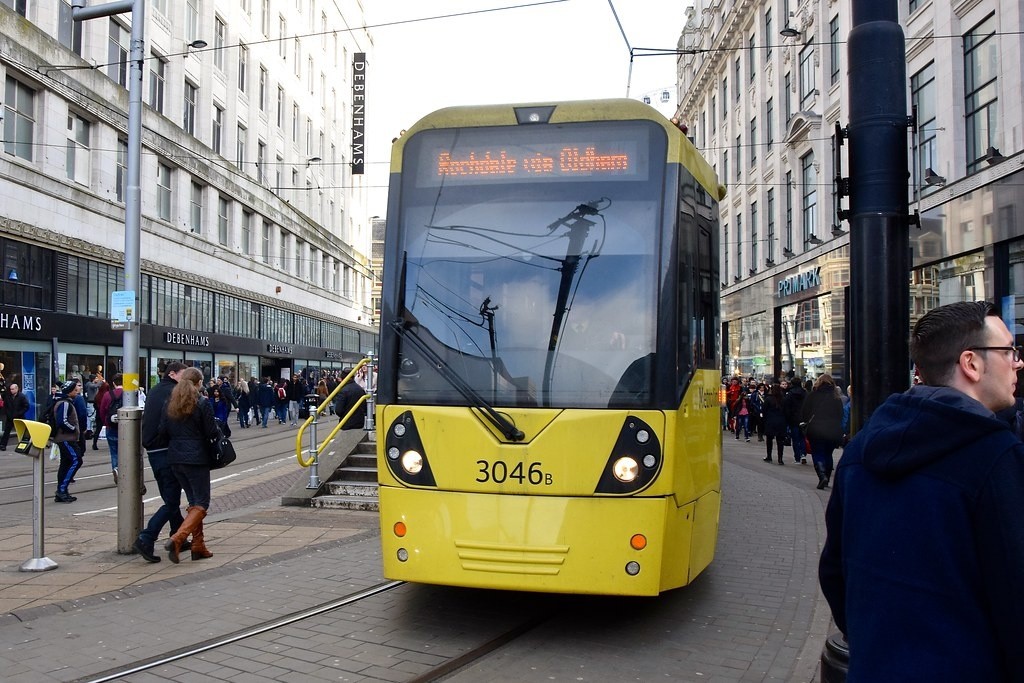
[340,367,355,378]
[89,374,96,382]
[54,381,63,388]
[60,381,76,394]
[217,376,224,381]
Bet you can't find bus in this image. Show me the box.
[377,97,729,596]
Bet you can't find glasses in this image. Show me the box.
[956,345,1022,364]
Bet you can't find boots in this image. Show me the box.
[186,506,213,561]
[164,507,207,564]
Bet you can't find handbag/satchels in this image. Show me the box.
[50,443,60,460]
[209,427,236,471]
[235,387,242,400]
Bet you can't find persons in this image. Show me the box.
[817,301,1024,683]
[720,368,851,490]
[133,360,225,564]
[92,381,110,450]
[199,372,340,427]
[332,367,367,431]
[67,364,105,401]
[0,363,8,400]
[0,384,29,452]
[540,300,626,351]
[354,360,378,427]
[99,372,123,485]
[47,378,88,504]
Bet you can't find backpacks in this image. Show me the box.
[275,384,286,399]
[107,390,123,430]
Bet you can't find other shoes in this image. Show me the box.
[54,495,77,502]
[726,424,831,489]
[131,537,161,562]
[180,541,192,552]
[236,406,336,428]
[113,469,118,484]
[0,445,6,451]
[69,478,75,483]
[92,443,99,450]
[55,490,73,503]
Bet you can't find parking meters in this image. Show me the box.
[13,419,60,572]
